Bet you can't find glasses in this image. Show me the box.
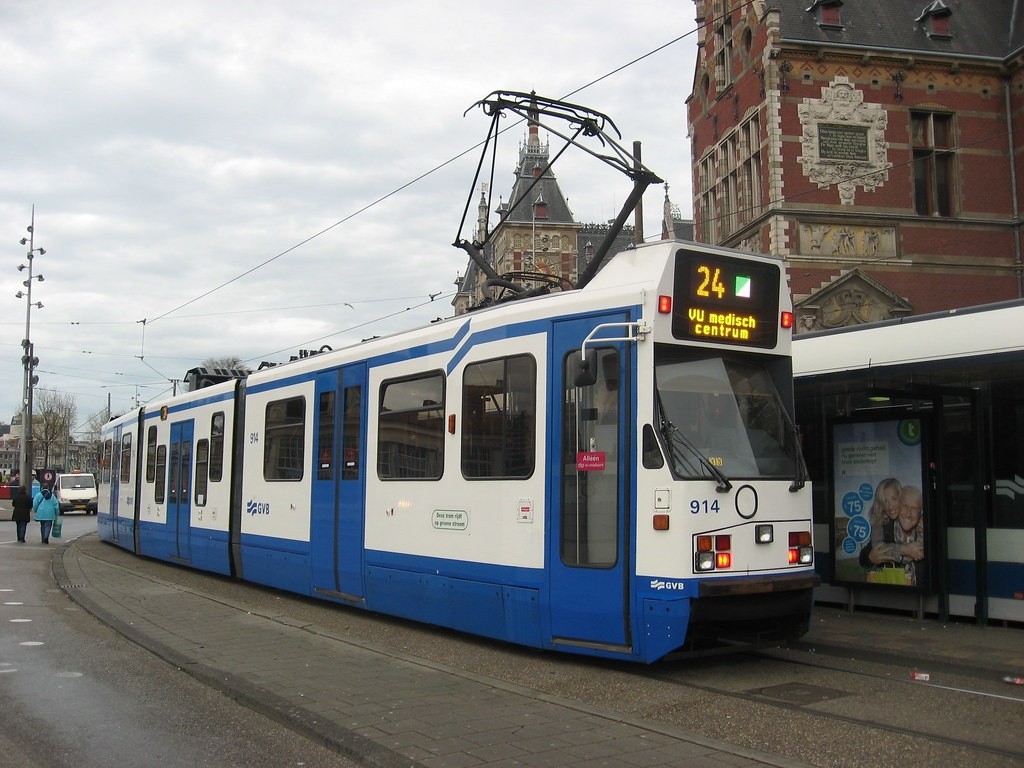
[899,504,922,514]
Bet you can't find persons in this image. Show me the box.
[858,477,924,578]
[33,483,60,544]
[9,474,41,504]
[11,485,34,543]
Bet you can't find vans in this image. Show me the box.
[53,474,99,515]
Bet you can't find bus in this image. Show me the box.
[100,87,817,666]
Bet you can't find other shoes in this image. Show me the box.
[44,538,49,544]
[18,538,20,542]
[21,538,25,542]
[41,537,44,543]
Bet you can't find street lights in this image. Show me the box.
[19,339,39,496]
[15,204,45,487]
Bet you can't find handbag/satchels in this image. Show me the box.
[52,520,63,538]
[866,562,914,586]
